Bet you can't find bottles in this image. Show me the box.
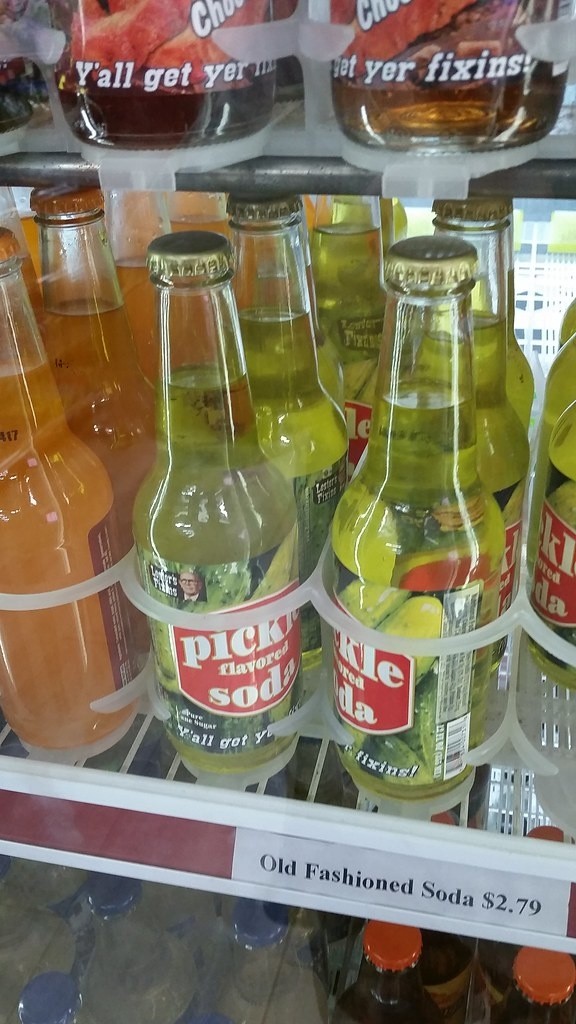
[0,0,576,1024]
